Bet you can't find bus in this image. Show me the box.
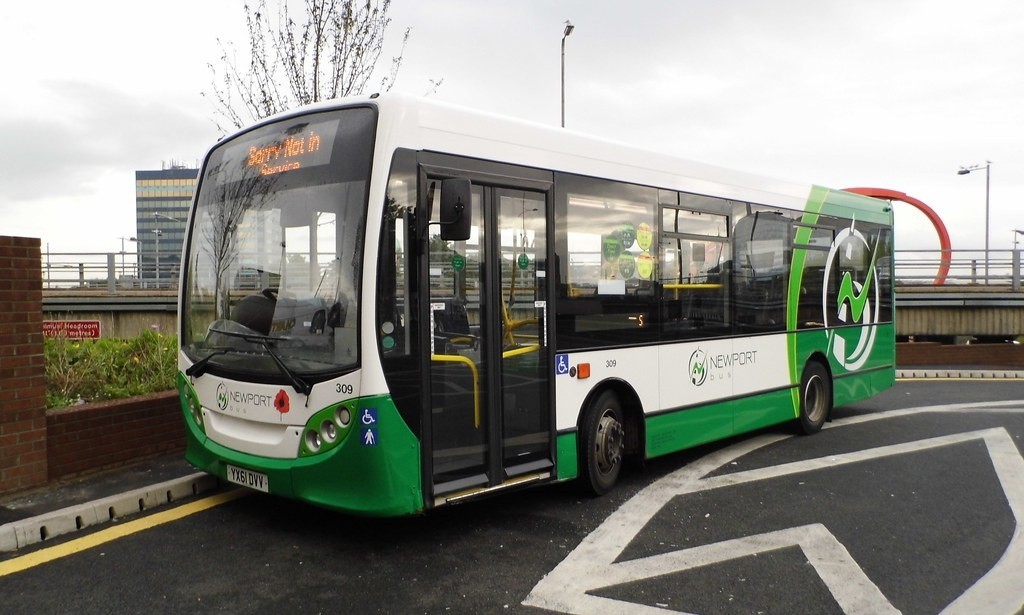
[174,96,897,515]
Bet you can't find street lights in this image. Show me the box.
[958,164,991,285]
[560,24,577,128]
